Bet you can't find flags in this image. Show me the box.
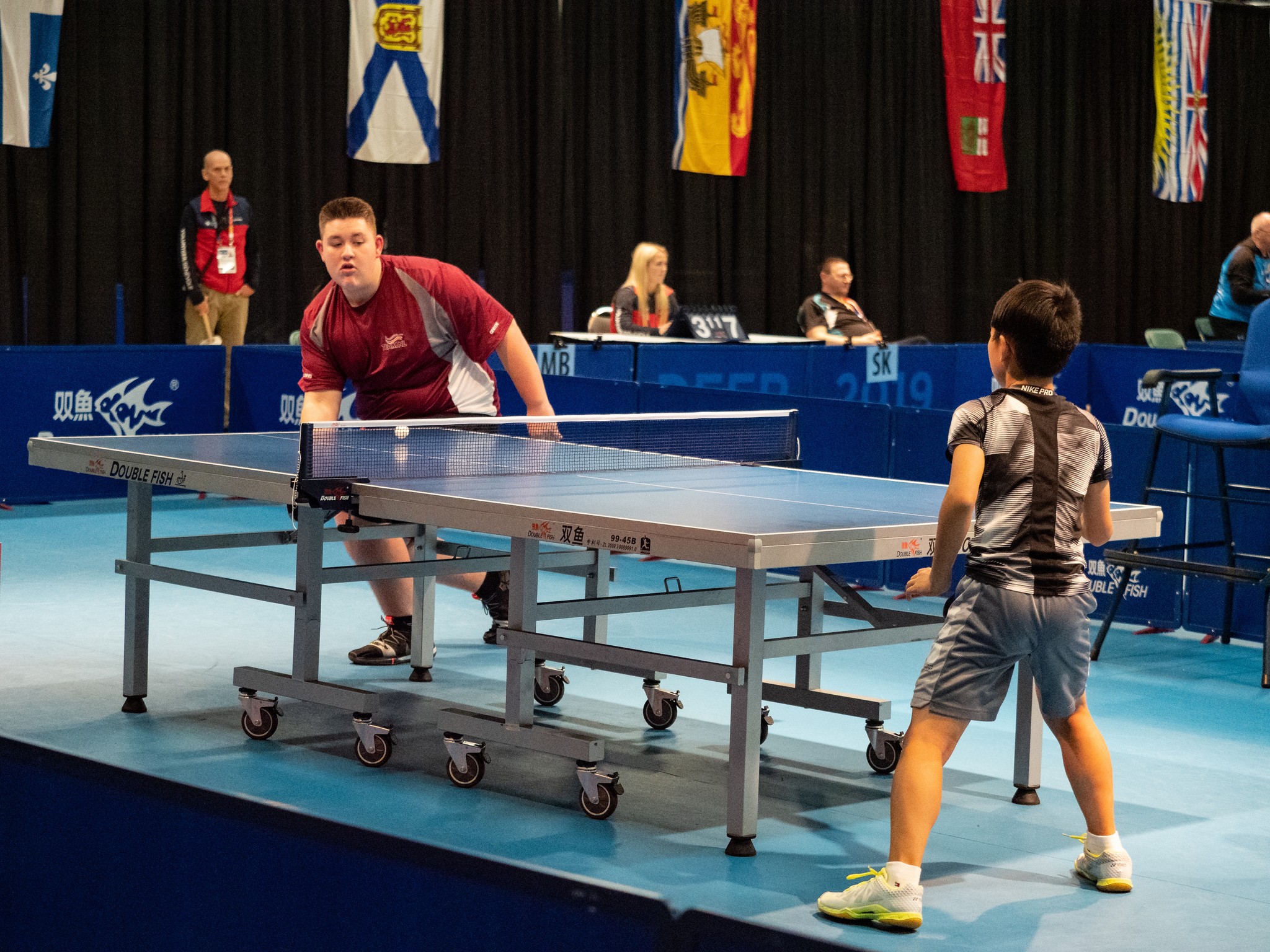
[1152,0,1212,202]
[346,0,444,163]
[941,0,1006,192]
[0,0,64,147]
[671,0,756,175]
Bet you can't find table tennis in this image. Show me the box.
[395,426,409,439]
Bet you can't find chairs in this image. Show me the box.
[1090,303,1270,687]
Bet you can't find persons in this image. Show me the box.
[1209,211,1270,341]
[298,197,562,665]
[610,242,679,336]
[818,279,1134,926]
[181,149,258,345]
[797,256,931,345]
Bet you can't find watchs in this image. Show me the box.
[843,336,852,345]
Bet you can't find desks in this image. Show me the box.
[27,408,1163,856]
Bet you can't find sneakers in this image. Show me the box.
[817,865,923,927]
[1060,831,1135,891]
[481,569,511,644]
[348,615,437,666]
